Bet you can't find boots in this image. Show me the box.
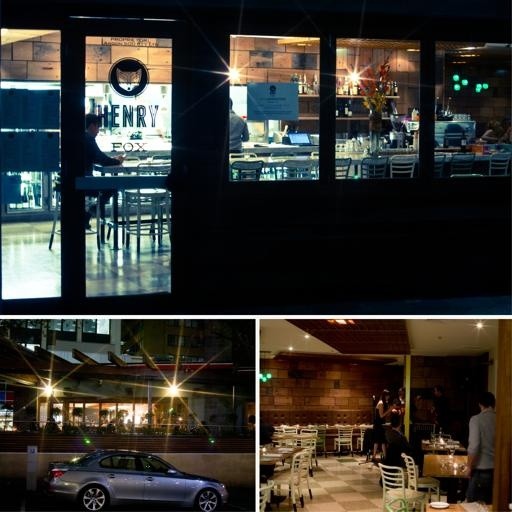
[84,211,92,229]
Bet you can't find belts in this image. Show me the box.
[480,469,493,473]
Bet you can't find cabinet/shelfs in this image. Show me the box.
[298,93,401,124]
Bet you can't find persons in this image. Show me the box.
[84,113,123,229]
[229,96,249,159]
[480,117,512,144]
[370,384,497,505]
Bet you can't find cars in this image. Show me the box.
[43,448,233,512]
[130,130,167,140]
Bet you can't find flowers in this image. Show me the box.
[348,47,402,146]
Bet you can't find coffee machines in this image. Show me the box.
[389,114,405,148]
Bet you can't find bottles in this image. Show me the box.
[291,73,398,96]
[411,108,419,121]
[335,100,352,118]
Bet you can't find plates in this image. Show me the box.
[430,501,449,508]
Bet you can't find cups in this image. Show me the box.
[444,112,472,121]
[456,498,490,511]
[341,130,384,153]
[147,158,152,164]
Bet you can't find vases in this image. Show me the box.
[366,108,382,154]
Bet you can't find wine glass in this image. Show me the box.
[430,432,455,463]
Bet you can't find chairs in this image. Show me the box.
[43,123,512,258]
[259,406,512,511]
